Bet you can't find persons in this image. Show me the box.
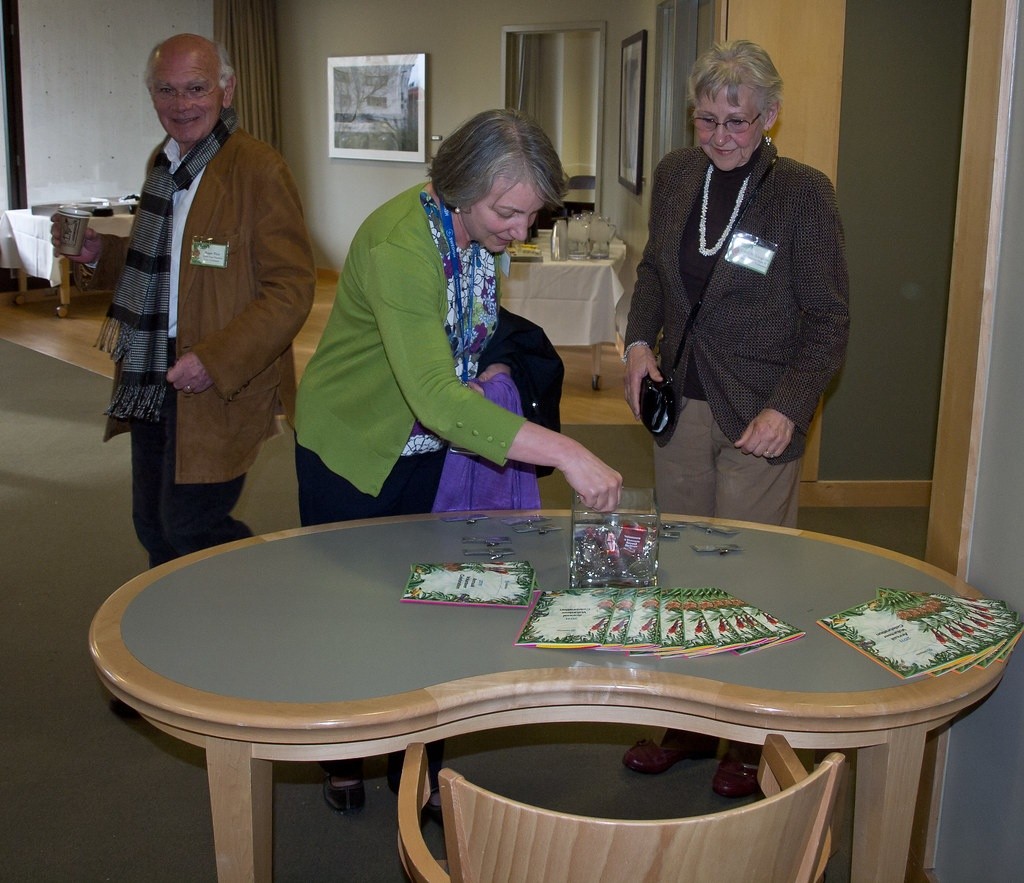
[624,41,852,801]
[51,32,315,720]
[292,109,624,826]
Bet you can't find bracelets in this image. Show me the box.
[624,342,650,359]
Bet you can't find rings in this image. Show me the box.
[187,385,191,390]
[766,450,772,456]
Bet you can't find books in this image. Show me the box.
[514,586,807,658]
[814,586,1023,680]
[400,561,541,608]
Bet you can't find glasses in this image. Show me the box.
[150,81,220,99]
[690,114,761,131]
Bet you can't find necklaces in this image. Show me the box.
[699,164,751,256]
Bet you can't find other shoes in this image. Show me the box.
[425,800,444,827]
[323,775,365,815]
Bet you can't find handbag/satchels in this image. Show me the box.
[638,365,678,437]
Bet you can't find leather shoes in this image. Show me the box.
[712,743,763,796]
[623,734,694,774]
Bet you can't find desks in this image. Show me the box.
[0,209,136,319]
[88,509,1012,883]
[499,228,628,391]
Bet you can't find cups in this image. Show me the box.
[551,210,616,261]
[57,205,92,255]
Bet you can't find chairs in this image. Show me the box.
[397,734,847,883]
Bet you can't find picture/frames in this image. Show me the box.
[327,51,426,164]
[618,30,648,194]
